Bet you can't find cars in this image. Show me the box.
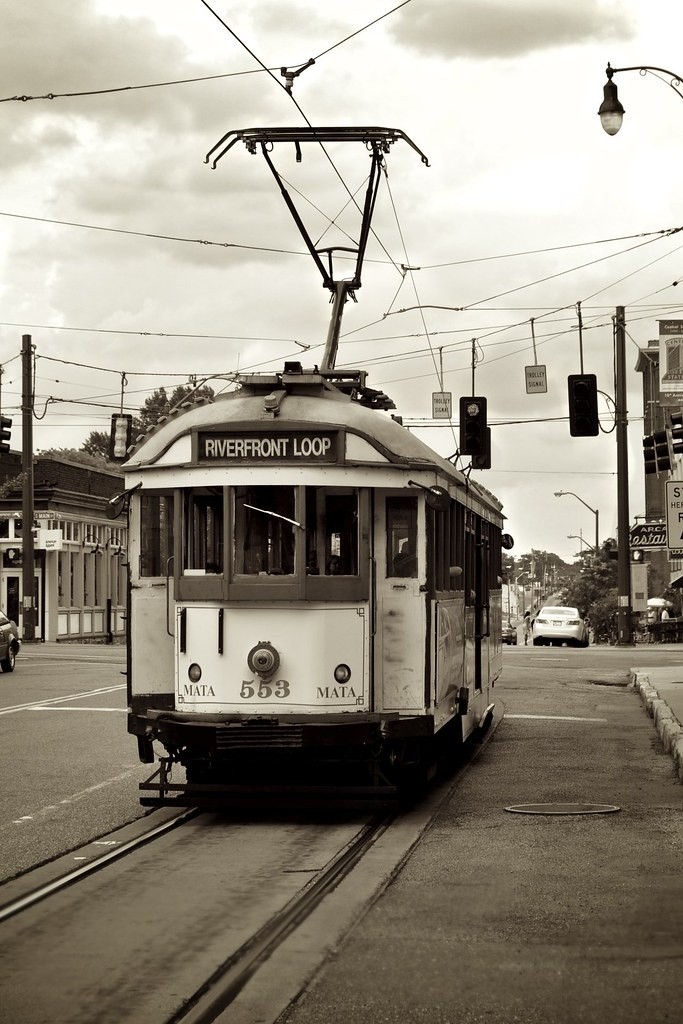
[0,610,20,672]
[502,621,519,645]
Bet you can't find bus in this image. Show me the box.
[121,121,514,808]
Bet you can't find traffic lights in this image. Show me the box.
[568,373,601,438]
[0,417,13,454]
[459,397,487,455]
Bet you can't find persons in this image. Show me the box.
[523,611,532,645]
[660,608,670,623]
[326,554,340,575]
[394,542,412,578]
[647,607,656,643]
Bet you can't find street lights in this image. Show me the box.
[554,491,600,557]
[530,605,591,648]
[566,535,597,567]
